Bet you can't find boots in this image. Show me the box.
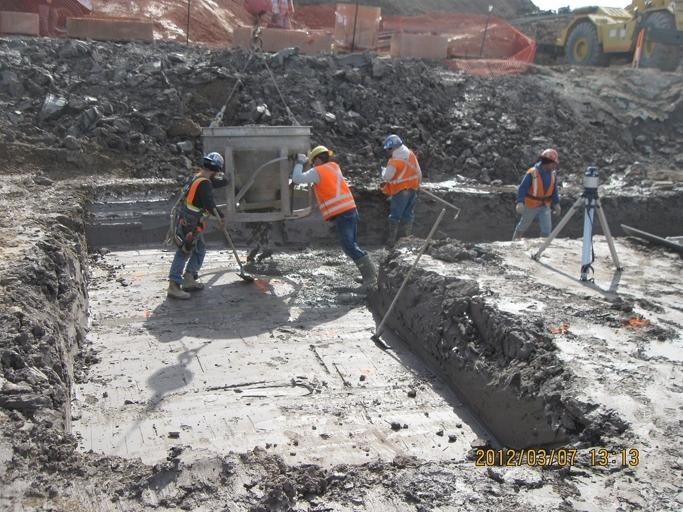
[382,223,412,248]
[168,281,191,299]
[354,253,379,293]
[182,273,204,291]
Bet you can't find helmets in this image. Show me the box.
[205,152,224,170]
[382,134,403,149]
[310,145,334,163]
[540,148,559,164]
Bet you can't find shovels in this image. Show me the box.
[370,208,447,351]
[213,206,255,282]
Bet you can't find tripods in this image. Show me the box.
[530,166,624,282]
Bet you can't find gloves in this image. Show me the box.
[553,203,561,215]
[515,202,524,213]
[298,154,309,163]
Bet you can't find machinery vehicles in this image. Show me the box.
[557,0,683,72]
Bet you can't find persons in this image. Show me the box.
[271,1,295,30]
[377,135,423,250]
[165,152,231,300]
[292,146,377,292]
[511,148,561,243]
[247,218,288,262]
